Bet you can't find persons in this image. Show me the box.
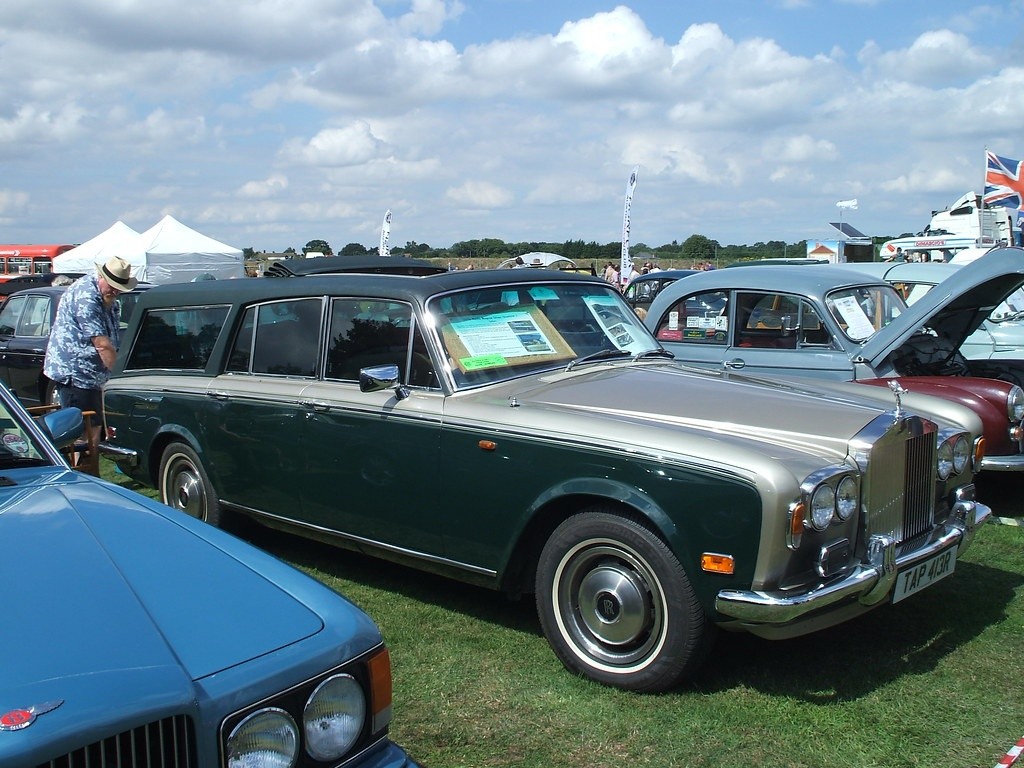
[455,264,474,270]
[884,247,954,263]
[507,257,525,269]
[688,261,716,272]
[601,260,678,299]
[196,269,263,282]
[43,255,138,476]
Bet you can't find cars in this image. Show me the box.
[0,273,88,310]
[98,256,992,693]
[645,241,1023,472]
[716,259,895,330]
[927,190,1024,247]
[624,271,727,324]
[0,284,160,412]
[950,244,998,266]
[1,380,421,768]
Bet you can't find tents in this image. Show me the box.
[50,214,244,285]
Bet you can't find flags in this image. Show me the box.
[620,166,640,284]
[983,152,1024,213]
[379,210,393,256]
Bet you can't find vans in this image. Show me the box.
[800,263,1023,361]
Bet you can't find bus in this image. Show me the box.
[880,234,996,263]
[0,243,77,283]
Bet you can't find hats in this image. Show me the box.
[530,258,544,265]
[93,256,138,293]
